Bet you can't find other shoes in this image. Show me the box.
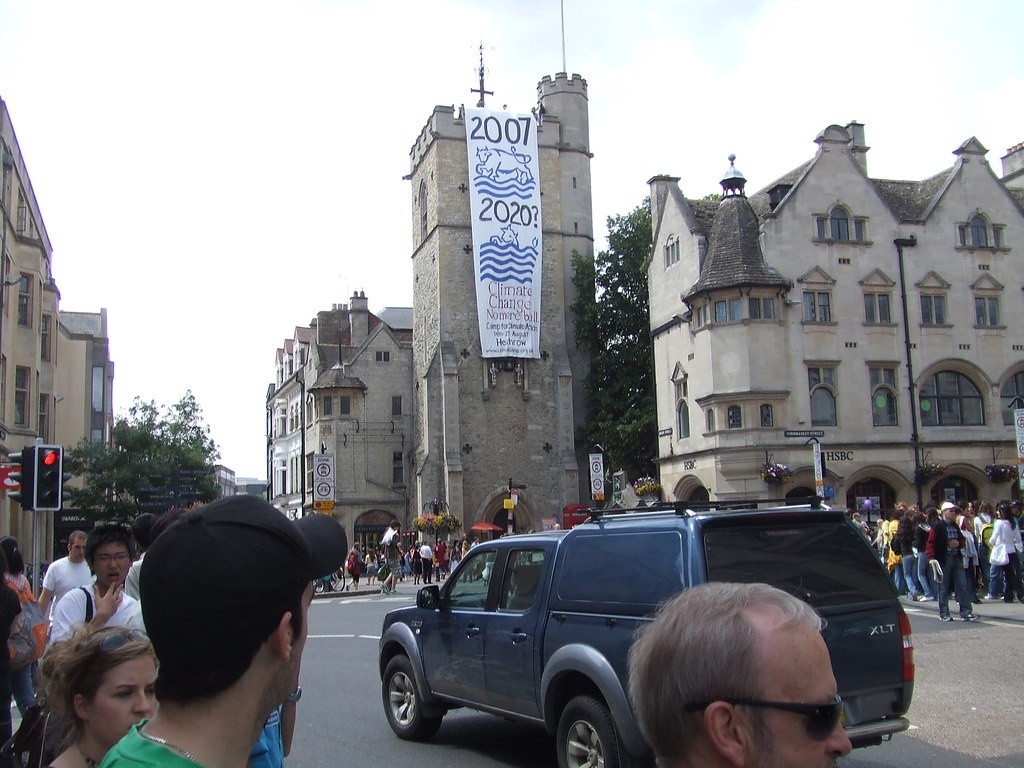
[939,613,953,623]
[391,591,401,595]
[380,583,389,595]
[443,572,447,579]
[1002,596,1015,604]
[346,584,349,591]
[962,612,982,622]
[919,594,936,603]
[985,593,1000,600]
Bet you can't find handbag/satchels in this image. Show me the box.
[380,527,398,547]
[374,554,379,570]
[989,542,1010,566]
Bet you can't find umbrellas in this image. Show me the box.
[471,518,505,542]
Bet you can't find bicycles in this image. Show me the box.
[313,568,345,594]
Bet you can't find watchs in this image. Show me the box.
[288,686,302,702]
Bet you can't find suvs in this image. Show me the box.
[378,493,916,767]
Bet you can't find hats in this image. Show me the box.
[138,495,348,665]
[940,502,958,512]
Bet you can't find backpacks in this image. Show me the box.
[5,578,48,672]
[978,514,994,548]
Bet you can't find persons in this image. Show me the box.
[925,500,981,624]
[43,624,158,768]
[101,495,347,768]
[625,580,852,768]
[38,531,92,646]
[47,524,149,659]
[317,519,494,594]
[847,501,1024,602]
[0,536,47,768]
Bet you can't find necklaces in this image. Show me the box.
[76,740,99,767]
[139,726,205,768]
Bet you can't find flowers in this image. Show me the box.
[760,462,793,483]
[985,463,1019,483]
[914,462,947,481]
[634,477,663,496]
[413,513,462,537]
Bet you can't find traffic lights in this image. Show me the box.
[35,445,61,512]
[7,450,24,506]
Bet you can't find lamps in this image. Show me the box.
[785,299,802,306]
[2,273,23,286]
[672,313,691,323]
[54,395,65,402]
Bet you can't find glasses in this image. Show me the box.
[684,692,843,739]
[96,554,130,564]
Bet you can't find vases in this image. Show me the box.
[992,475,1011,483]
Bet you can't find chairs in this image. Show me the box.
[507,565,542,610]
